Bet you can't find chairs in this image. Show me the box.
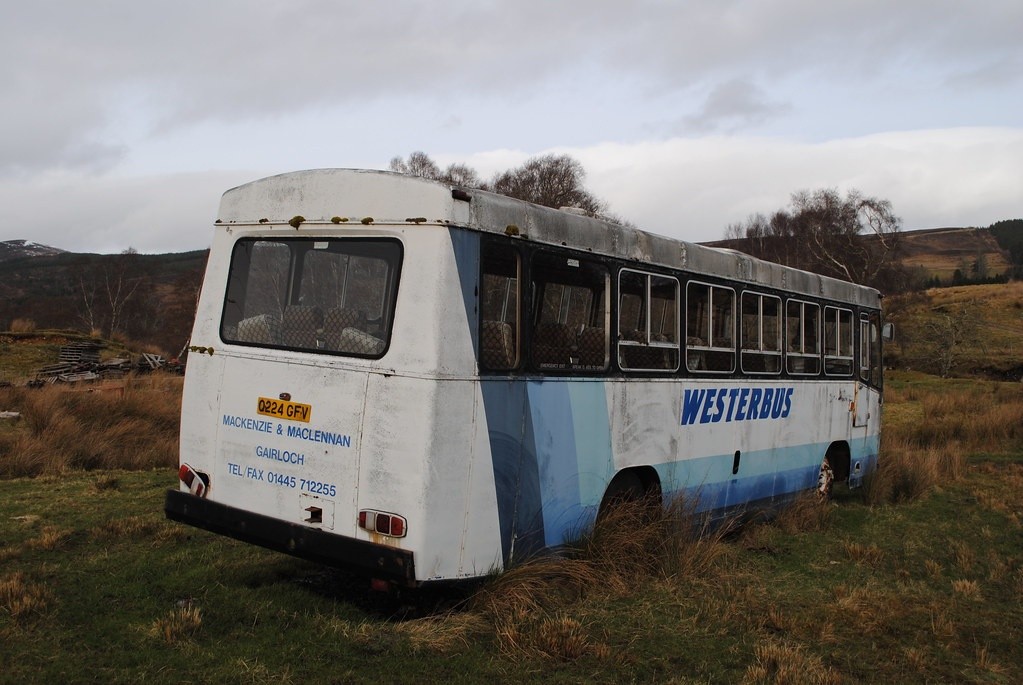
[650,332,672,368]
[624,328,656,368]
[792,335,816,373]
[578,327,628,368]
[687,337,707,370]
[482,320,515,369]
[766,343,795,372]
[532,325,578,364]
[702,338,731,371]
[322,307,367,351]
[337,328,386,355]
[237,313,282,345]
[743,342,766,372]
[281,305,325,350]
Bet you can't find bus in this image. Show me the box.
[164,166,896,597]
